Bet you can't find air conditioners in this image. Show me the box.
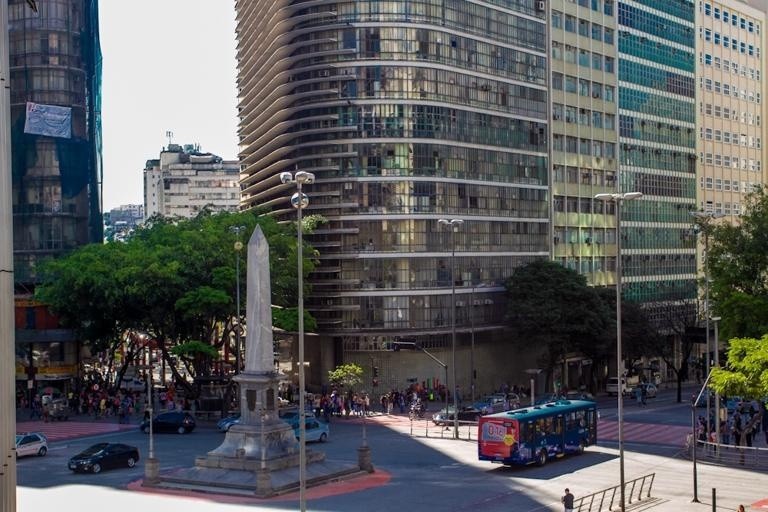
[588,237,593,244]
[484,299,494,305]
[474,300,482,305]
[536,0,546,11]
[456,300,465,307]
[600,284,607,289]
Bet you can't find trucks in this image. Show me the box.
[606,376,640,397]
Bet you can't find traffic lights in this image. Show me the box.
[373,367,379,377]
[387,342,392,350]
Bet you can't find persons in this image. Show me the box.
[561,488,574,512]
[636,388,643,409]
[17,349,192,426]
[641,387,649,409]
[277,380,538,422]
[695,401,765,448]
[737,504,745,512]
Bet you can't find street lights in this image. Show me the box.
[438,215,464,438]
[230,226,246,372]
[594,192,644,512]
[281,171,316,511]
[688,210,726,424]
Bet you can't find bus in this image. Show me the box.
[478,399,601,468]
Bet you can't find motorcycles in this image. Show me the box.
[409,405,425,420]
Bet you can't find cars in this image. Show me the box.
[141,411,196,434]
[68,442,140,473]
[690,390,715,408]
[726,395,759,413]
[215,412,242,433]
[631,384,657,398]
[16,431,49,459]
[280,411,330,444]
[432,393,520,426]
